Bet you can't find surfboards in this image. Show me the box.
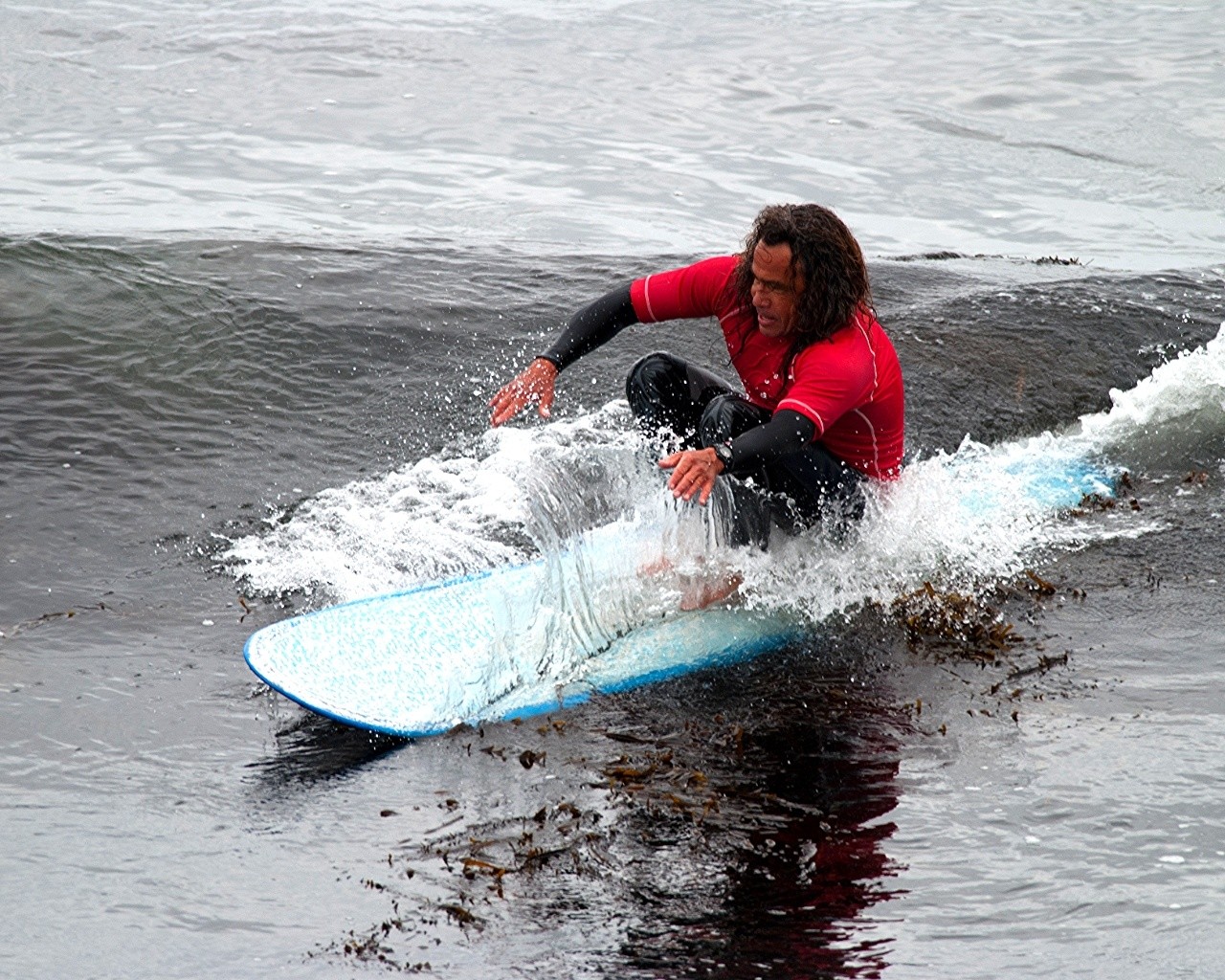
[242,457,1117,740]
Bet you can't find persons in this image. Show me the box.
[487,203,905,608]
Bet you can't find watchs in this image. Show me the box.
[712,443,733,471]
